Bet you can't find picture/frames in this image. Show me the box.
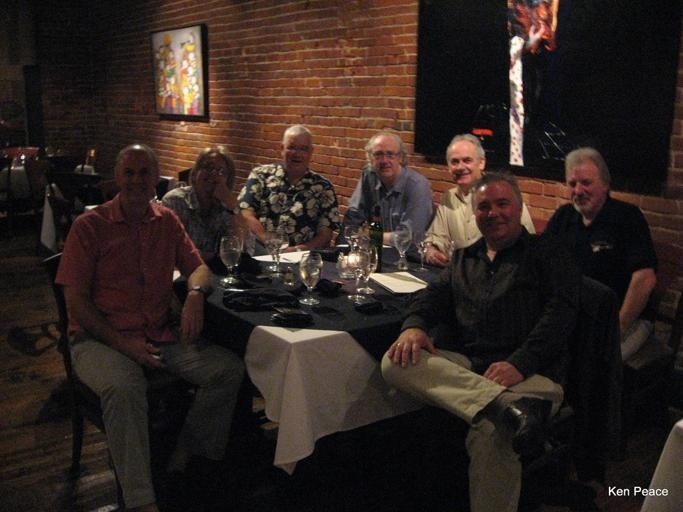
[148,21,208,122]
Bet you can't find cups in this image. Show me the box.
[11,157,17,169]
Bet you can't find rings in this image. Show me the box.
[395,342,401,350]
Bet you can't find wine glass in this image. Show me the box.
[392,223,413,271]
[218,235,242,284]
[413,228,433,273]
[298,252,323,306]
[334,224,379,305]
[442,238,456,266]
[263,229,290,278]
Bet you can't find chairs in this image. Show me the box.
[2,139,189,236]
[626,289,678,425]
[43,248,215,512]
[498,277,624,512]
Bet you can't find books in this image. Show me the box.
[369,270,429,294]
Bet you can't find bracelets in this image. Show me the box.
[294,245,301,252]
[185,283,209,294]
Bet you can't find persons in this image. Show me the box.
[54,144,212,512]
[342,131,435,248]
[238,124,340,256]
[415,131,539,268]
[379,173,563,511]
[542,148,657,360]
[441,1,631,176]
[160,145,244,272]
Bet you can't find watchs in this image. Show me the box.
[225,204,241,216]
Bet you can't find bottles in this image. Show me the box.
[368,205,384,272]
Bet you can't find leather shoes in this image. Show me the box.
[504,395,553,451]
